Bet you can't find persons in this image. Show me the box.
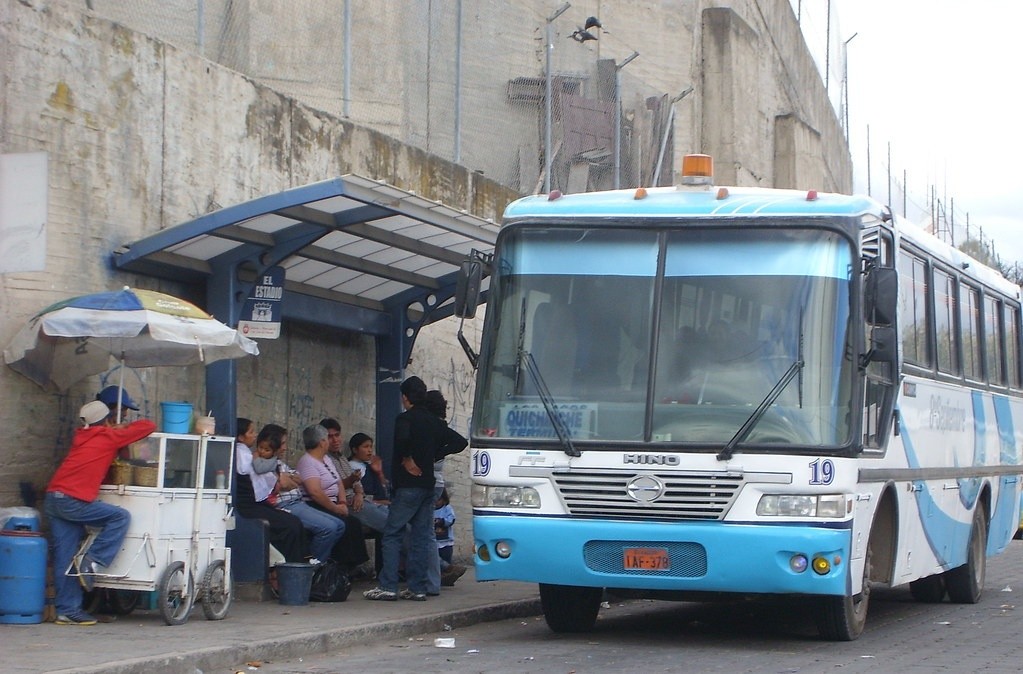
[662,320,766,385]
[363,376,468,601]
[434,488,456,565]
[253,431,282,507]
[44,386,156,625]
[236,390,447,598]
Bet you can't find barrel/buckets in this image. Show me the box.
[160,400,194,434]
[102,457,160,486]
[276,564,313,605]
[175,469,192,487]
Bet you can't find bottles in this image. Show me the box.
[215,470,225,489]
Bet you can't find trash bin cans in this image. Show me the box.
[275,561,316,606]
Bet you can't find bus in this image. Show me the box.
[453,154,1023,641]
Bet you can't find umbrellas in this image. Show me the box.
[3,286,261,424]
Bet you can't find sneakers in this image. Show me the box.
[55,609,97,625]
[74,553,96,591]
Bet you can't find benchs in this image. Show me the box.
[235,514,370,602]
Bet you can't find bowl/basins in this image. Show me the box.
[195,416,215,434]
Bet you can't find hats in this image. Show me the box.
[79,401,111,429]
[99,386,139,411]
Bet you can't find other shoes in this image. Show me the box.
[440,564,467,586]
[399,587,426,601]
[363,585,397,601]
[268,567,279,597]
